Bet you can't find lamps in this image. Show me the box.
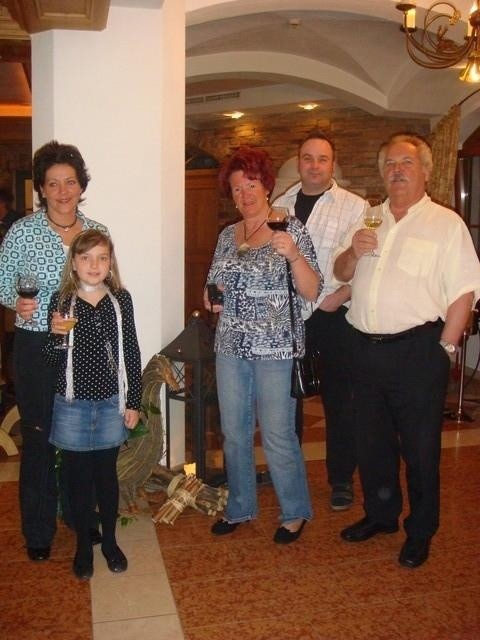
[395,0,479,86]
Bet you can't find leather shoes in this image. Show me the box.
[398,536,429,569]
[341,516,399,542]
[101,544,128,573]
[210,475,228,489]
[211,518,241,535]
[273,518,307,544]
[26,545,51,561]
[73,545,94,579]
[88,528,102,546]
[255,470,274,485]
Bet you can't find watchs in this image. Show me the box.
[439,338,456,356]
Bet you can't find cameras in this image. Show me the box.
[207,282,224,305]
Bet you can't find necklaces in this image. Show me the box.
[237,212,271,255]
[47,211,77,232]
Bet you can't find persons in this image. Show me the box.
[39,229,142,577]
[0,140,117,562]
[271,129,371,513]
[333,130,480,568]
[0,172,21,385]
[209,146,324,543]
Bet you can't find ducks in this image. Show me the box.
[116,352,181,515]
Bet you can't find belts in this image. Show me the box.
[352,319,442,345]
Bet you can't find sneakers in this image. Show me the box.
[329,482,355,511]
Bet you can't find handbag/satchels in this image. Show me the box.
[289,355,321,400]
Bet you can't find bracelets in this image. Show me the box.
[287,254,301,264]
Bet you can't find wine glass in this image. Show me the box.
[362,198,383,258]
[265,206,289,260]
[53,304,78,351]
[15,274,40,326]
